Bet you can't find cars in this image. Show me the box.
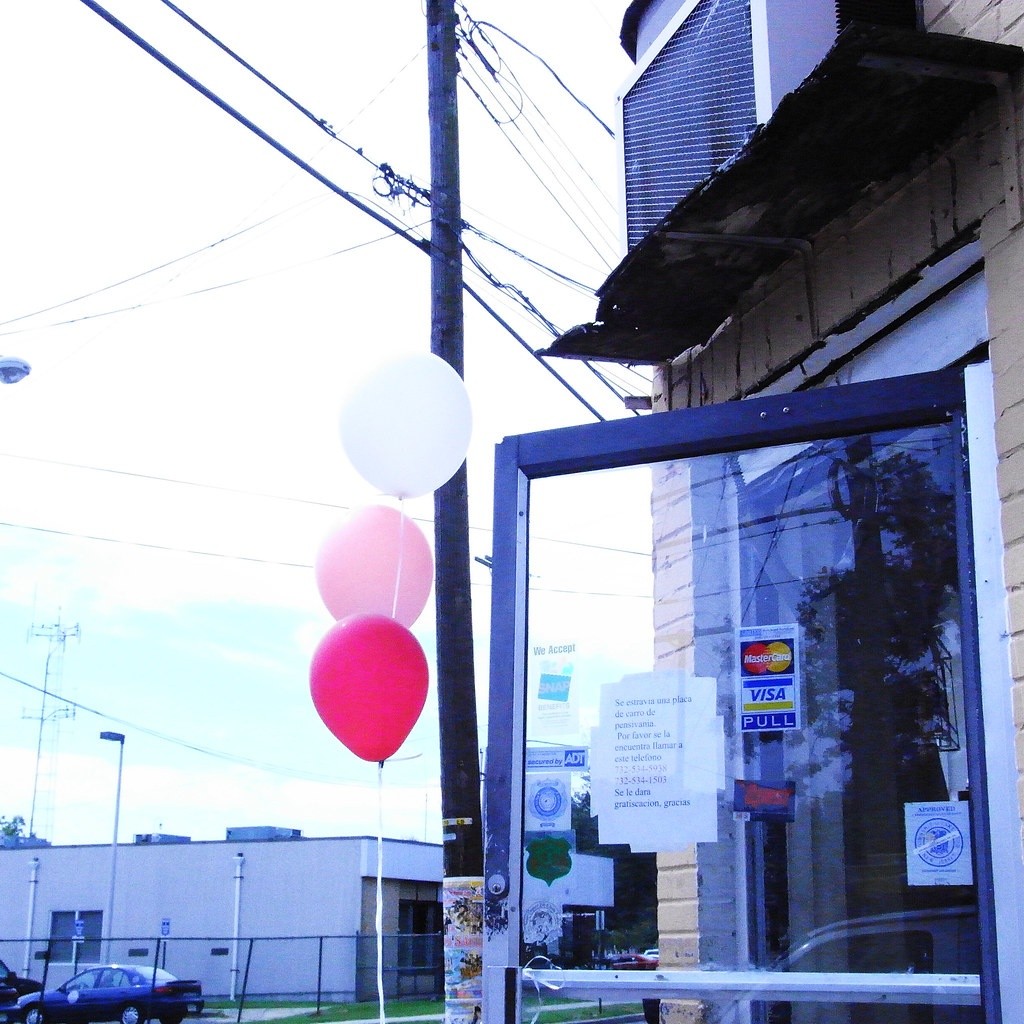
[648,955,659,961]
[15,963,205,1023]
[600,954,658,972]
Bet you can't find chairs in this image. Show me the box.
[101,975,114,987]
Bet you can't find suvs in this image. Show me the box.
[0,958,43,1024]
[709,908,982,1022]
[644,949,659,956]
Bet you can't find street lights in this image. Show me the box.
[0,354,31,385]
[97,728,125,962]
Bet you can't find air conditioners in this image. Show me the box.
[616,0,841,261]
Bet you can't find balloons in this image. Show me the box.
[339,346,473,500]
[318,505,433,628]
[309,612,429,768]
